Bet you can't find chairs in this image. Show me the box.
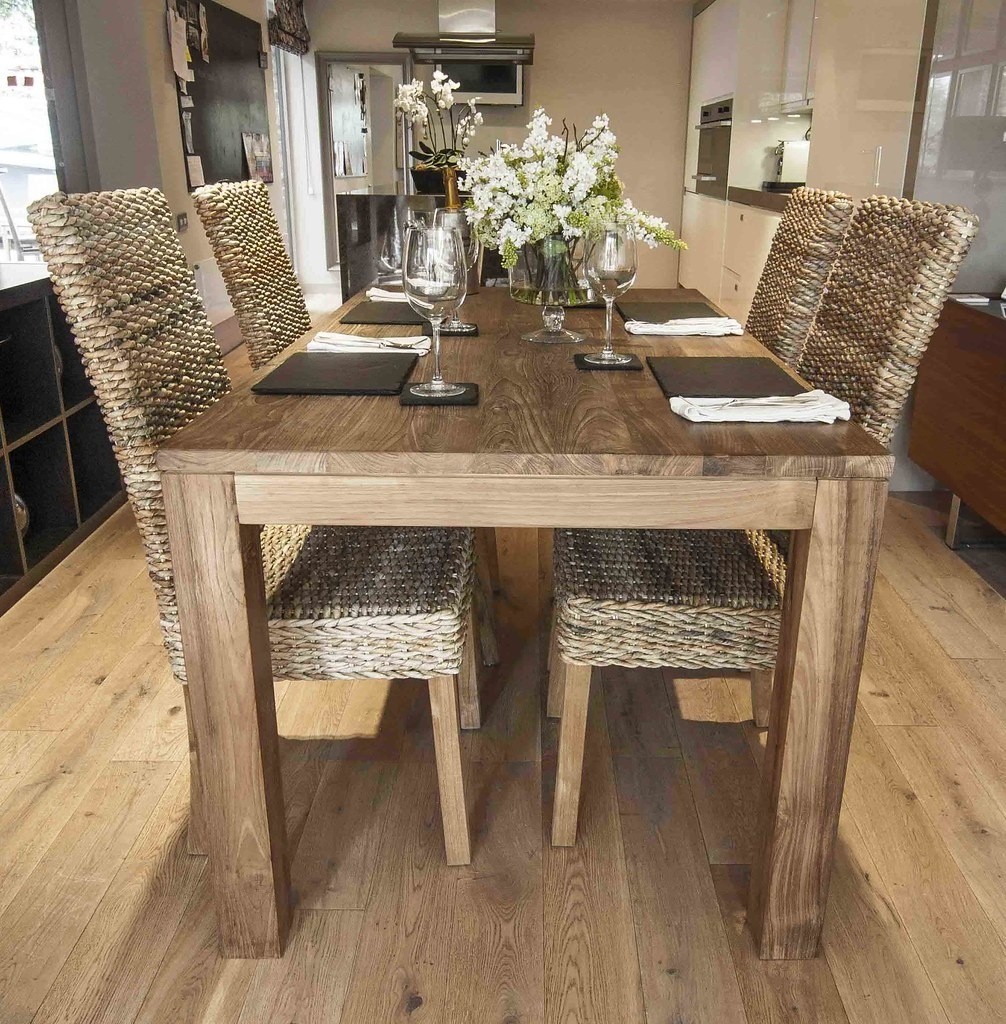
[741,188,851,363]
[549,195,975,848]
[27,188,486,866]
[191,176,499,668]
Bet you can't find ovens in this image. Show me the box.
[688,97,733,200]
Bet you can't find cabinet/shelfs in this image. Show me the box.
[0,268,127,614]
[781,0,815,113]
[720,202,781,331]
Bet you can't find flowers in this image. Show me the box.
[456,106,684,259]
[395,69,484,167]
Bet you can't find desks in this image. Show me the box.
[908,291,1006,551]
[159,288,895,961]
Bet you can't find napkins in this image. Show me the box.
[669,387,852,424]
[307,331,432,357]
[365,288,408,303]
[623,317,745,337]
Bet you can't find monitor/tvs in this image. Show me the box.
[435,47,523,105]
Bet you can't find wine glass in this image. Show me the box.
[581,223,638,365]
[431,207,479,333]
[400,224,468,397]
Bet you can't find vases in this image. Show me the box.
[411,166,473,194]
[504,229,605,306]
